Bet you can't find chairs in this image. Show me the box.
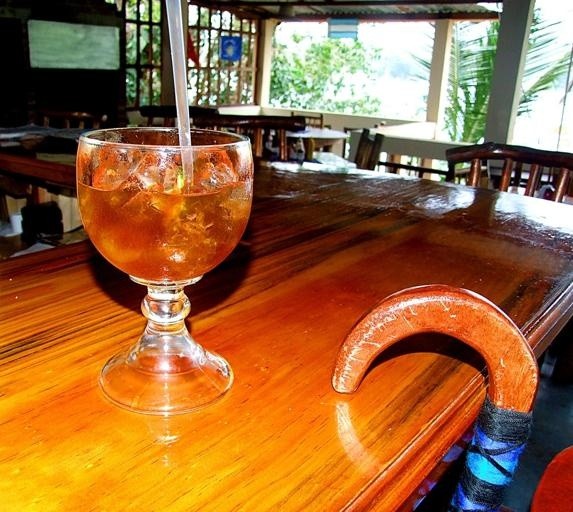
[32,109,114,133]
[140,107,180,129]
[192,106,573,202]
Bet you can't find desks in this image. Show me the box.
[0,175,573,512]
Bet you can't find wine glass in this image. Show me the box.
[75,127,254,416]
